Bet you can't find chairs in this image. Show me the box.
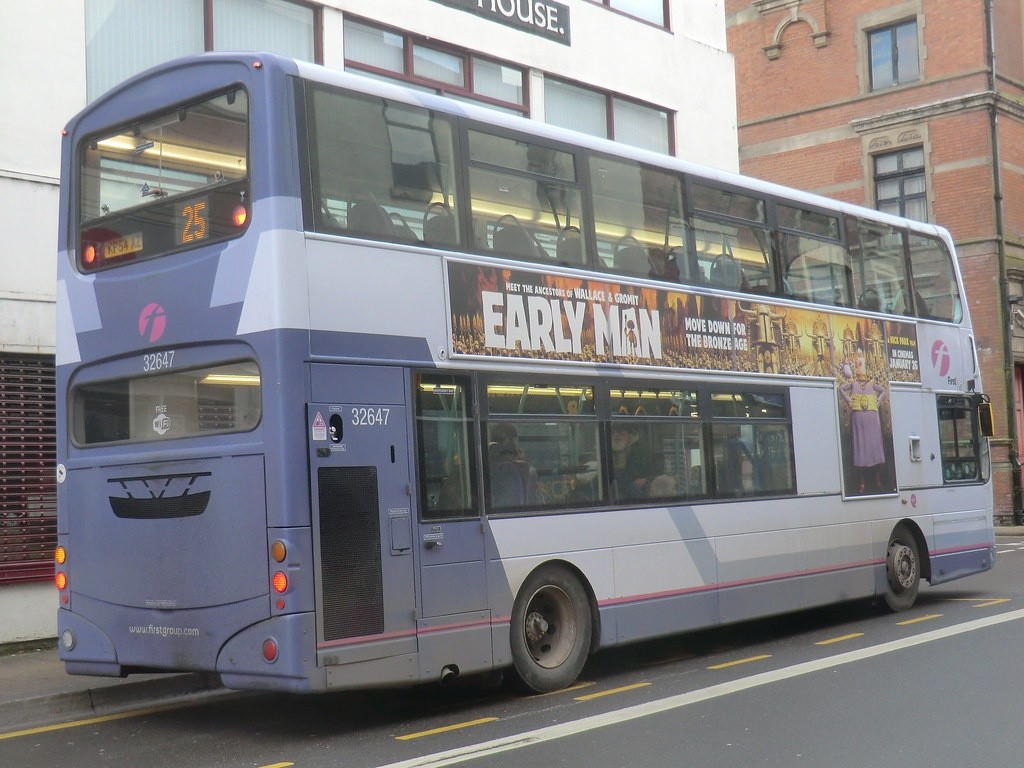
[489,441,538,510]
[320,183,932,319]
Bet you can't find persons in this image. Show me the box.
[488,423,552,504]
[649,475,676,499]
[610,421,658,500]
[650,248,666,274]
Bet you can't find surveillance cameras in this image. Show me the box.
[1013,309,1024,320]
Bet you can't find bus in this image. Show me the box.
[60,49,994,694]
